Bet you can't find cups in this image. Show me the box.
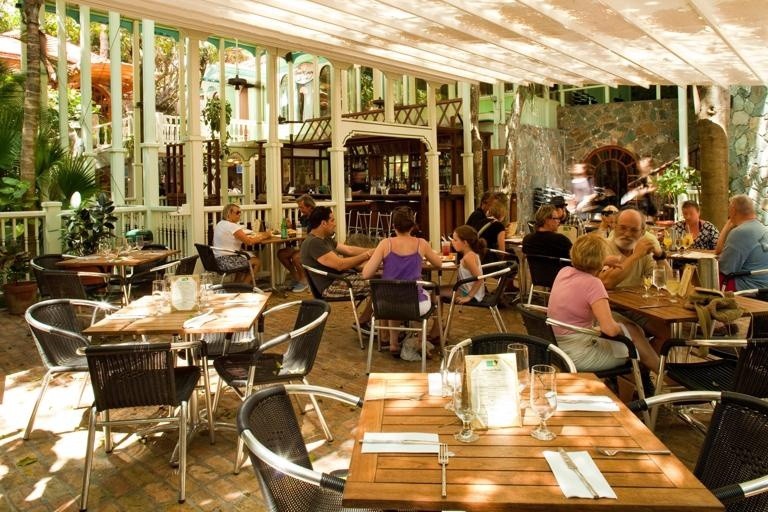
[441,241,451,257]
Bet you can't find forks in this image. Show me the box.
[591,441,671,457]
[437,443,449,497]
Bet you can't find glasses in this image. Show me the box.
[232,210,242,215]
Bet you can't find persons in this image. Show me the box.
[212,203,271,282]
[277,194,316,293]
[350,170,365,203]
[428,226,487,342]
[363,206,442,360]
[301,207,378,338]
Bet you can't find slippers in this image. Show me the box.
[391,347,401,357]
[417,349,433,360]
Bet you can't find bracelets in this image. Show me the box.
[366,250,372,260]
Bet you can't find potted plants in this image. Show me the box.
[0,237,40,317]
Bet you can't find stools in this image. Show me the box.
[346,200,419,237]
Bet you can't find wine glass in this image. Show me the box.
[101,232,145,261]
[152,271,214,315]
[642,264,680,303]
[440,340,561,443]
[662,227,694,255]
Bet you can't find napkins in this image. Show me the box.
[360,432,441,455]
[108,312,148,318]
[542,451,617,500]
[428,372,462,396]
[183,314,218,330]
[547,393,619,412]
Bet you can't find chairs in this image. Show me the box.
[236,384,363,512]
[23,298,121,440]
[196,229,768,434]
[624,391,768,512]
[440,332,577,373]
[30,245,198,299]
[75,340,202,512]
[204,297,333,443]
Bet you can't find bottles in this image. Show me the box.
[280,216,303,239]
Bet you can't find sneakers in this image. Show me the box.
[289,280,309,293]
[350,321,379,339]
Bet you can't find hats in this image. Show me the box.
[545,196,568,206]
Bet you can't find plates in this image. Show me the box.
[440,256,456,262]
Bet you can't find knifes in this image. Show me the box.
[358,439,441,445]
[639,304,674,309]
[558,446,600,499]
[559,398,614,405]
